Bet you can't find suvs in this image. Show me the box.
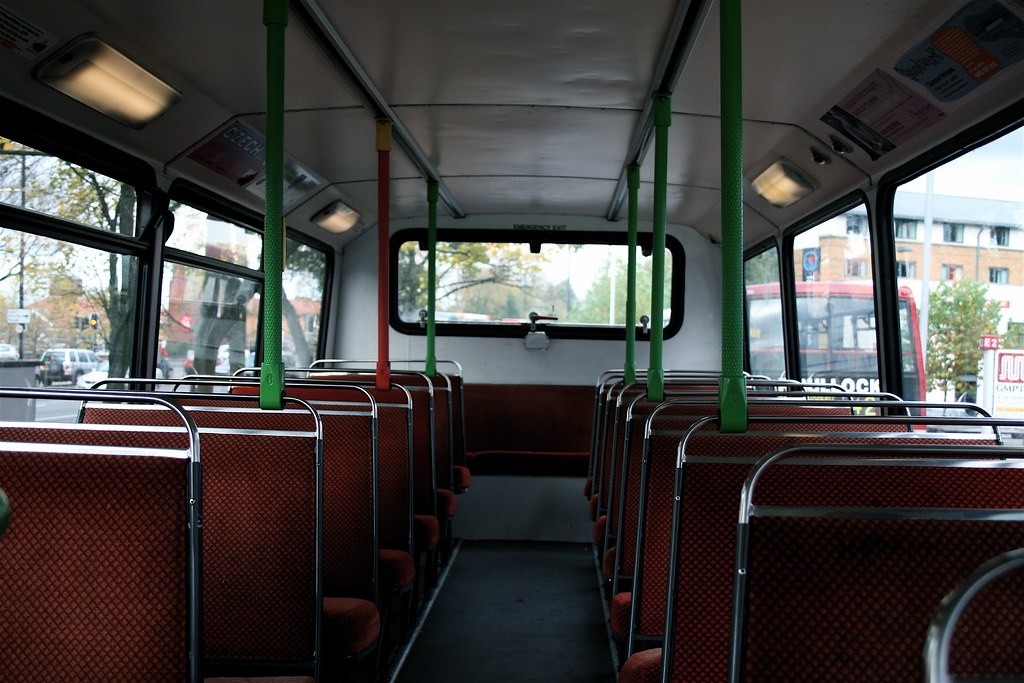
[38,348,103,388]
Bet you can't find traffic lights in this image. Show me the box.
[90,312,98,330]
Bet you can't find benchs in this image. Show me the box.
[0,357,1024,683]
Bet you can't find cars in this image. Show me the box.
[0,344,21,363]
[184,344,251,379]
[76,359,164,394]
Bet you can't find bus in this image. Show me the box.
[747,280,927,435]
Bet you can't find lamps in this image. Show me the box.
[750,155,817,209]
[308,199,360,234]
[27,29,183,131]
